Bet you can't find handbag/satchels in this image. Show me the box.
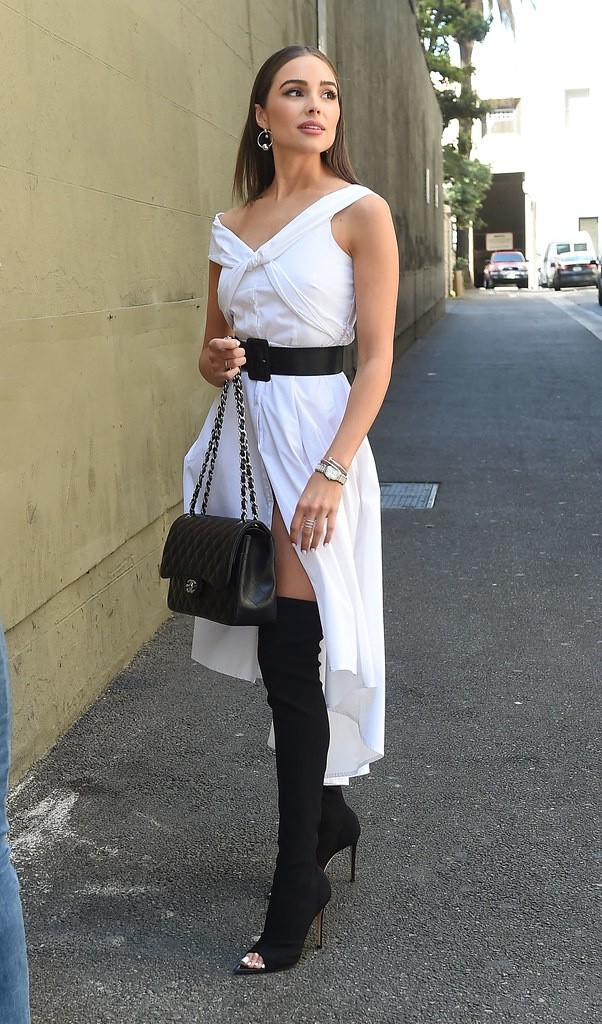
[161,513,278,627]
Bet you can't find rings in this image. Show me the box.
[304,518,314,529]
[224,359,228,367]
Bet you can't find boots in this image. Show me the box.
[267,785,361,898]
[233,595,332,972]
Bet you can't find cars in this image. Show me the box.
[550,250,599,291]
[590,253,602,308]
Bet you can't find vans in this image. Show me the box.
[538,230,597,289]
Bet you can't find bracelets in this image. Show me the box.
[321,457,347,476]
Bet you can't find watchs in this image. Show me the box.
[315,462,347,485]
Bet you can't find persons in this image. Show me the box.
[184,45,400,974]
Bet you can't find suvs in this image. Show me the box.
[483,251,531,289]
